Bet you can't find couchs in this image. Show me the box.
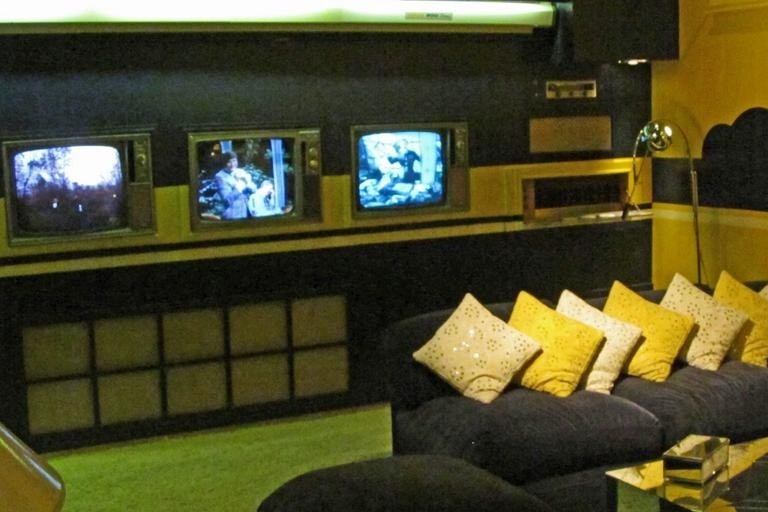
[256,453,554,511]
[387,278,767,508]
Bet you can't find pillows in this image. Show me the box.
[658,272,749,372]
[600,280,696,383]
[501,289,604,399]
[553,288,643,397]
[757,283,767,297]
[710,269,767,368]
[410,292,541,406]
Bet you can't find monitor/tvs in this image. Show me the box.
[1,130,158,248]
[350,122,470,219]
[187,128,323,231]
